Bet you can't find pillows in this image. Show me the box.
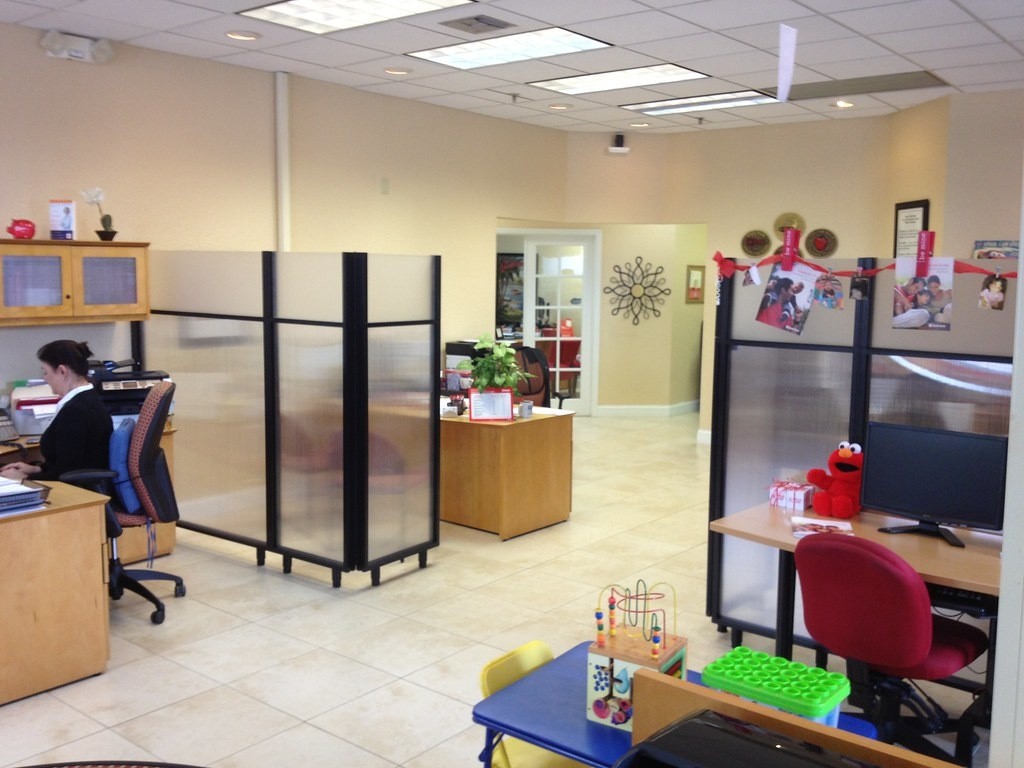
[110,418,143,512]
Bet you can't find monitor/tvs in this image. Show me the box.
[860,421,1009,549]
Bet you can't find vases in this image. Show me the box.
[96,230,117,241]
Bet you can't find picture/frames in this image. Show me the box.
[685,265,705,304]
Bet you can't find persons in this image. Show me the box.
[980,279,1005,310]
[822,281,839,306]
[757,277,804,331]
[892,275,951,331]
[0,339,127,512]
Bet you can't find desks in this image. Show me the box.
[0,415,178,708]
[320,395,575,539]
[473,637,877,768]
[707,490,1002,730]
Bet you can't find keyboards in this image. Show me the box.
[926,582,989,615]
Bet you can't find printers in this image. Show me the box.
[94,369,170,416]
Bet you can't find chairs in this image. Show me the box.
[511,346,549,409]
[482,639,598,767]
[274,416,422,546]
[788,532,988,764]
[104,379,188,625]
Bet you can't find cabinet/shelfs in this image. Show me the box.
[0,239,151,329]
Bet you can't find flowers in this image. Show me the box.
[79,181,115,231]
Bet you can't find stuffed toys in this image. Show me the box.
[806,440,864,520]
